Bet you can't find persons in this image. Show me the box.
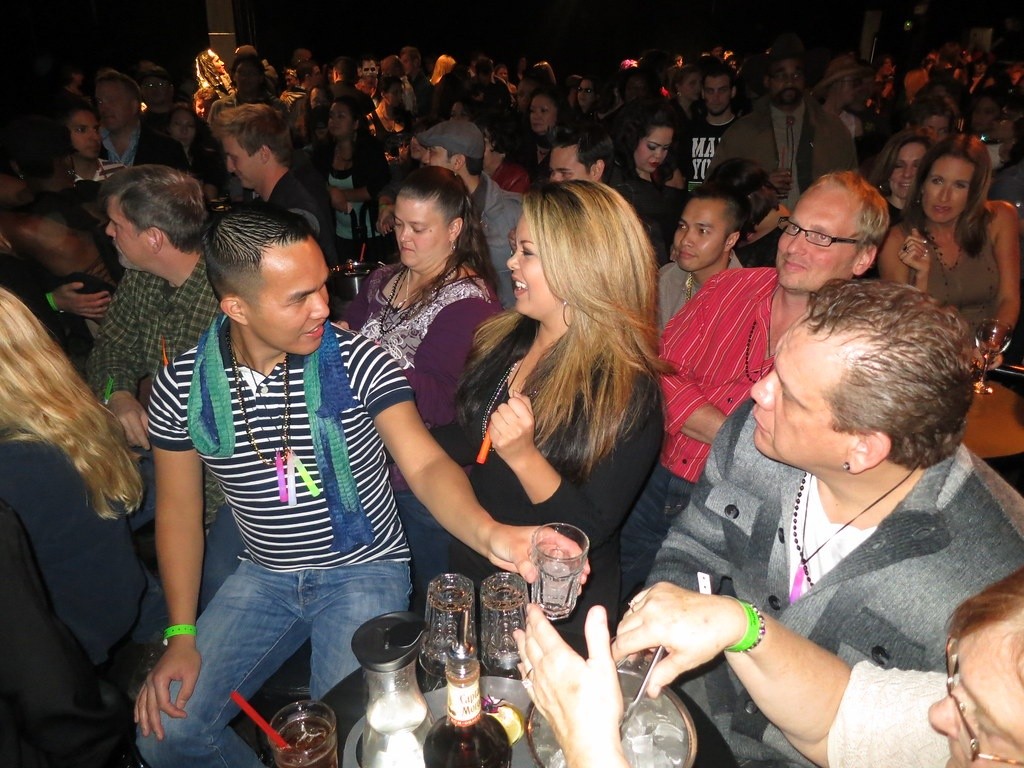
[79,164,244,617]
[645,279,1024,767]
[134,201,593,768]
[511,566,1024,768]
[0,288,172,768]
[616,172,889,607]
[401,180,666,659]
[0,0,1024,371]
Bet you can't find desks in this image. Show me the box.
[317,629,741,767]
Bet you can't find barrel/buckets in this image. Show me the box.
[329,262,377,299]
[523,668,698,768]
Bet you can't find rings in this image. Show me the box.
[522,668,534,689]
[903,244,911,253]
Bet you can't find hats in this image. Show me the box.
[742,33,830,93]
[416,118,485,159]
[7,120,80,163]
[813,55,872,96]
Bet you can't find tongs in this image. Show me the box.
[614,646,665,742]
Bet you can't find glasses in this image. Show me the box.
[763,71,805,81]
[364,68,375,71]
[776,215,865,247]
[143,80,167,91]
[945,636,1024,766]
[576,87,593,94]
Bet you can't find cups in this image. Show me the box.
[532,523,590,621]
[419,572,478,678]
[479,571,530,679]
[773,168,792,196]
[267,700,338,768]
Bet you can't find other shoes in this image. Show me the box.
[123,635,169,697]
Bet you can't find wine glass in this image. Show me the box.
[971,318,1012,396]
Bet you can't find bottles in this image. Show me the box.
[350,612,436,768]
[422,640,512,768]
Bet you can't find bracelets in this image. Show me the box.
[162,625,197,646]
[724,594,765,653]
[101,378,118,406]
[46,292,65,314]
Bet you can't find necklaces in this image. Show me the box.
[791,462,919,606]
[225,328,320,507]
[745,319,758,385]
[929,232,962,271]
[685,273,694,302]
[475,358,538,464]
[375,266,460,344]
